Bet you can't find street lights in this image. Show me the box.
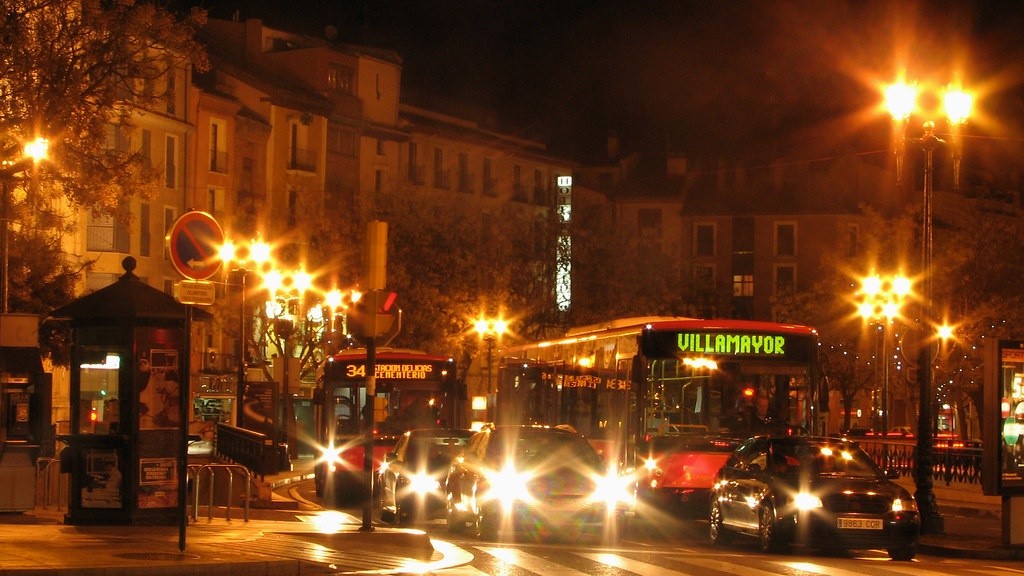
[473,312,510,423]
[3,138,53,312]
[885,78,974,536]
[260,267,317,472]
[215,240,270,430]
[856,271,913,436]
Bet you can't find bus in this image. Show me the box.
[496,317,830,532]
[310,347,470,508]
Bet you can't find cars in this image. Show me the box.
[444,422,631,546]
[378,428,476,526]
[707,435,922,562]
[890,427,912,435]
